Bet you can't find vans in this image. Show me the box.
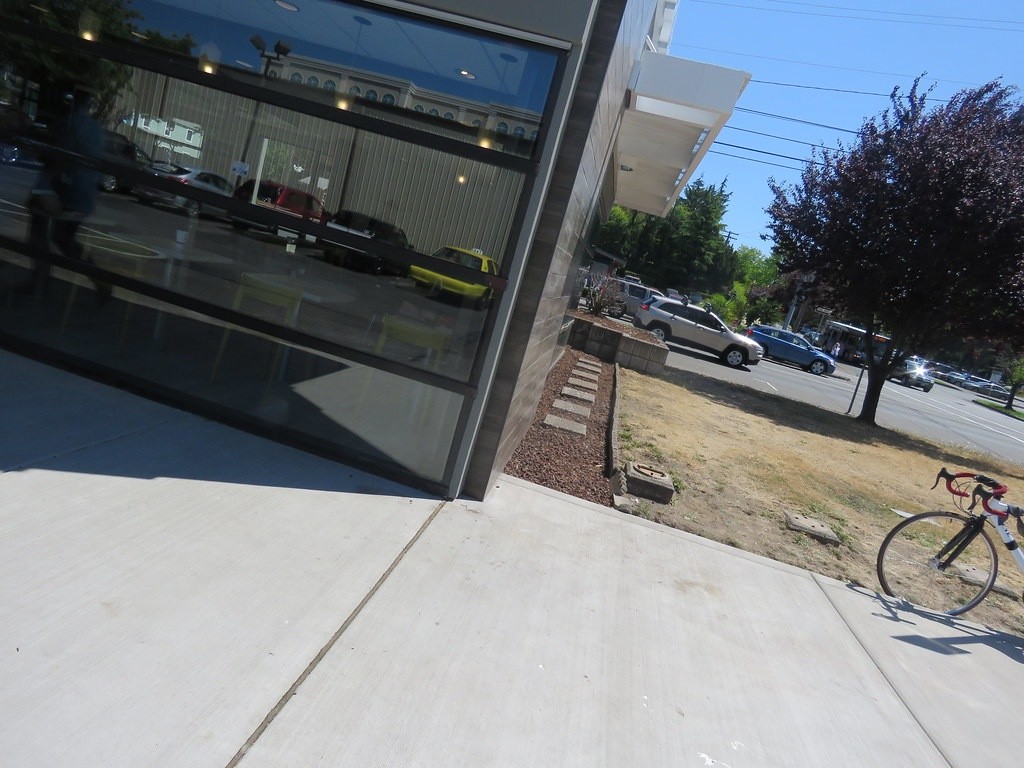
[227,179,330,241]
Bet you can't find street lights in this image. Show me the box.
[234,33,293,189]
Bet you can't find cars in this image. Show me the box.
[134,159,237,222]
[885,359,935,393]
[0,99,153,195]
[742,324,837,378]
[632,294,764,369]
[316,210,418,278]
[804,332,821,346]
[579,266,688,320]
[891,349,1011,402]
[407,245,500,312]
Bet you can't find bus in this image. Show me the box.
[816,318,891,369]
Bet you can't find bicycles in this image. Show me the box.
[877,466,1024,616]
